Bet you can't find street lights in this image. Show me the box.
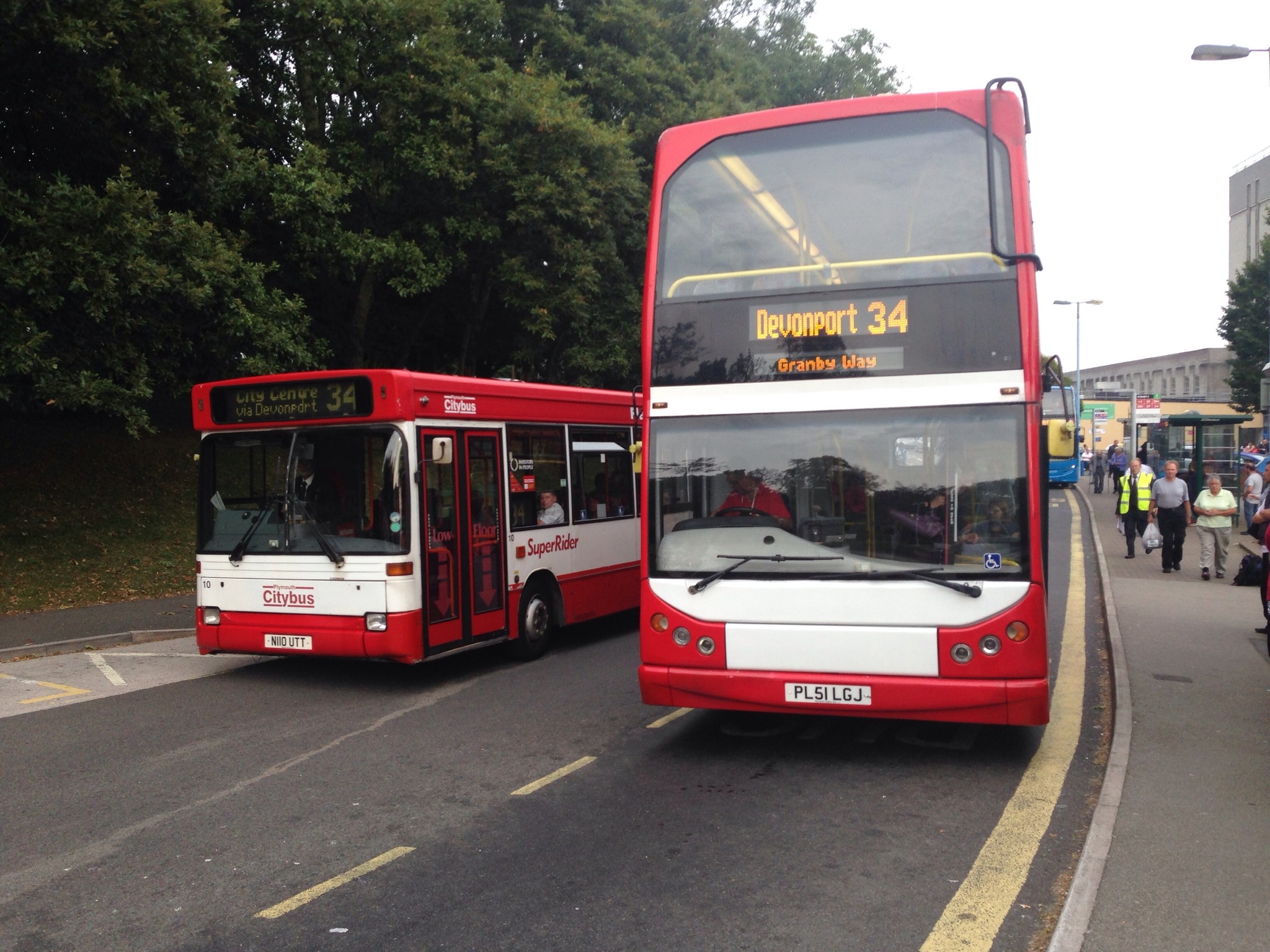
[1054,299,1103,479]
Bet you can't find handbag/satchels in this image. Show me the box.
[1142,522,1164,549]
[1116,518,1125,536]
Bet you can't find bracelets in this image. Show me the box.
[927,500,931,509]
[1147,513,1153,515]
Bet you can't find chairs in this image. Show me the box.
[699,453,1023,562]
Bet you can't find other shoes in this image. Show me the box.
[1256,625,1270,633]
[1146,549,1152,554]
[1240,528,1251,535]
[1094,491,1097,493]
[1098,491,1102,493]
[1125,555,1135,559]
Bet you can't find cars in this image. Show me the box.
[1168,443,1270,475]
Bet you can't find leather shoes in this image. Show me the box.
[1172,563,1181,570]
[1163,568,1171,573]
[1201,567,1210,580]
[1216,573,1224,578]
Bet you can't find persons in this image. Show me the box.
[535,491,566,527]
[830,454,1021,565]
[281,452,340,531]
[1081,439,1270,657]
[708,465,794,531]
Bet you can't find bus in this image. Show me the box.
[1042,385,1087,489]
[627,77,1075,727]
[191,368,732,670]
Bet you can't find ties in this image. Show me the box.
[1133,476,1137,486]
